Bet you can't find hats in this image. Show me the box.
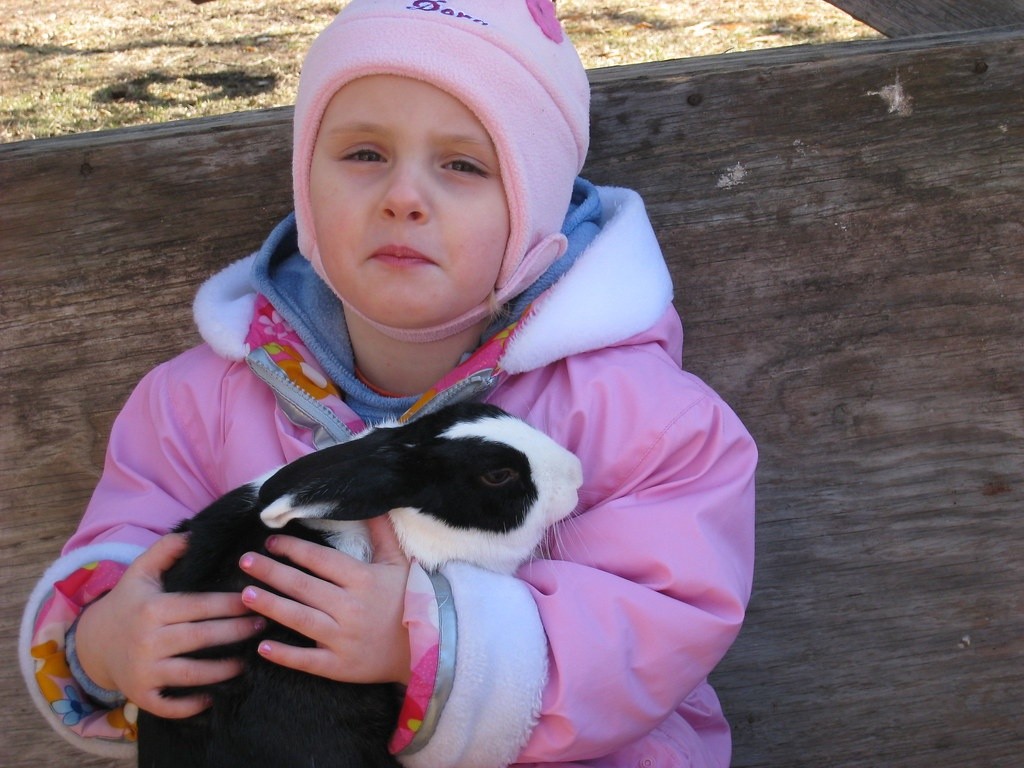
[292,0,591,343]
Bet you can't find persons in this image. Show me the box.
[19,0,759,768]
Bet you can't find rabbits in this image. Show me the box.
[134,400,587,768]
